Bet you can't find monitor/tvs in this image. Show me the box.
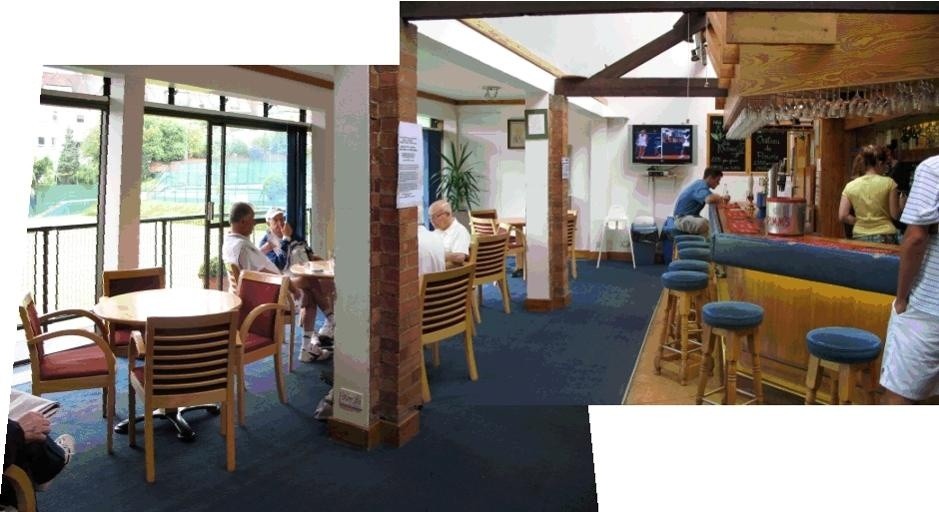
[626,120,698,176]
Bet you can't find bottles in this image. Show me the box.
[901,121,939,149]
[720,184,729,211]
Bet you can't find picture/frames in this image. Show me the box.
[506,119,526,150]
[524,108,549,140]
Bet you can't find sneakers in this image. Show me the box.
[318,316,335,342]
[297,344,330,363]
[32,434,77,494]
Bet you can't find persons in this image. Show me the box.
[259,207,336,325]
[879,154,939,404]
[636,129,648,157]
[673,167,730,234]
[838,145,906,245]
[427,199,471,266]
[681,128,690,158]
[321,224,445,387]
[0,412,76,512]
[222,203,335,362]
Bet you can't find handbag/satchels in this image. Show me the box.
[289,245,310,265]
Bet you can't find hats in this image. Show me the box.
[265,207,284,223]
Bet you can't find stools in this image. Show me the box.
[693,301,767,404]
[653,234,717,387]
[803,325,883,405]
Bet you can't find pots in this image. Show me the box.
[765,195,807,237]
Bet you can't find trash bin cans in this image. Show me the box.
[631,222,658,265]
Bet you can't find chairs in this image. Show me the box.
[19,293,117,455]
[234,269,291,426]
[222,208,579,403]
[128,310,236,484]
[99,268,163,417]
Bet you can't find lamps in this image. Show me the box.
[690,46,700,63]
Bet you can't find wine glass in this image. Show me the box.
[748,80,939,121]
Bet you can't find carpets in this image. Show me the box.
[1,253,671,512]
[1,207,581,511]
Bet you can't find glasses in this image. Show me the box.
[428,212,446,220]
